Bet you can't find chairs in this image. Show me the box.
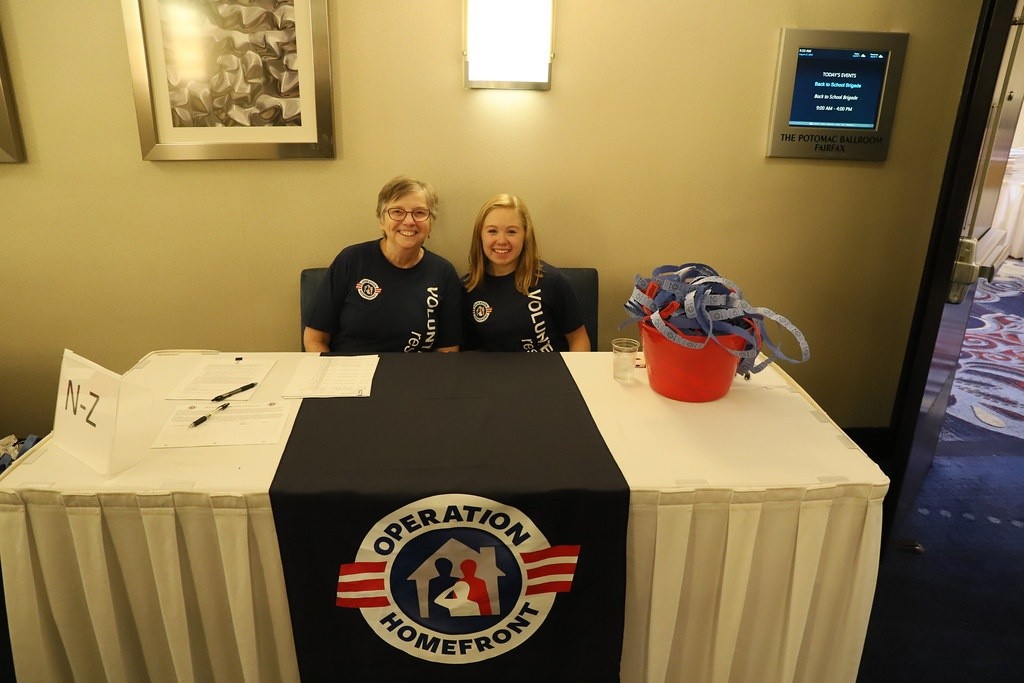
[302,267,599,351]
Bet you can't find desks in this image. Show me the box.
[1,350,891,683]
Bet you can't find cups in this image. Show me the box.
[611,337,640,383]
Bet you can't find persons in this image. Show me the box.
[459,197,591,351]
[302,176,466,352]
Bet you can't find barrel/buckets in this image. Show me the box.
[636,321,756,403]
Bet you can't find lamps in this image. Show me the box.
[462,1,553,90]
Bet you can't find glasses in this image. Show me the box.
[384,207,435,222]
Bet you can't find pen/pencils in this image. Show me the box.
[211,383,258,401]
[188,402,230,428]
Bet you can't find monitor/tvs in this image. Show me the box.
[766,27,910,161]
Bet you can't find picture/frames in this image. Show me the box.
[121,0,335,162]
